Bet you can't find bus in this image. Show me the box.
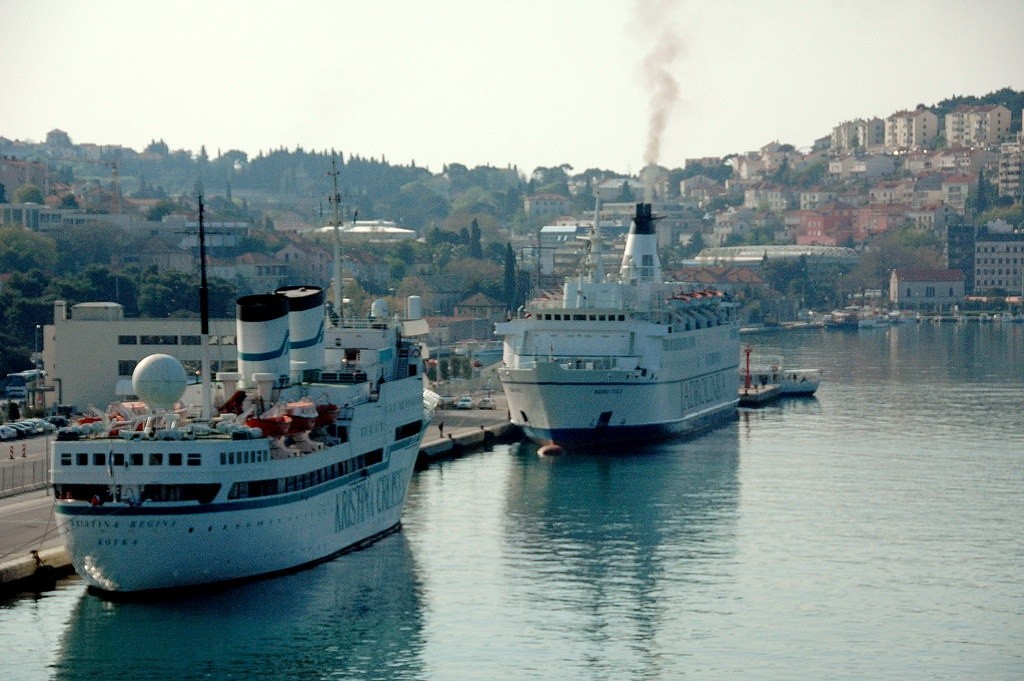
[3,369,45,398]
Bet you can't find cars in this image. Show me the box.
[479,396,495,409]
[44,416,67,428]
[457,397,475,409]
[0,419,55,441]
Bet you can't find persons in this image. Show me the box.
[438,420,444,437]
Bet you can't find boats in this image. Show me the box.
[45,156,443,598]
[488,202,749,452]
[744,365,823,394]
